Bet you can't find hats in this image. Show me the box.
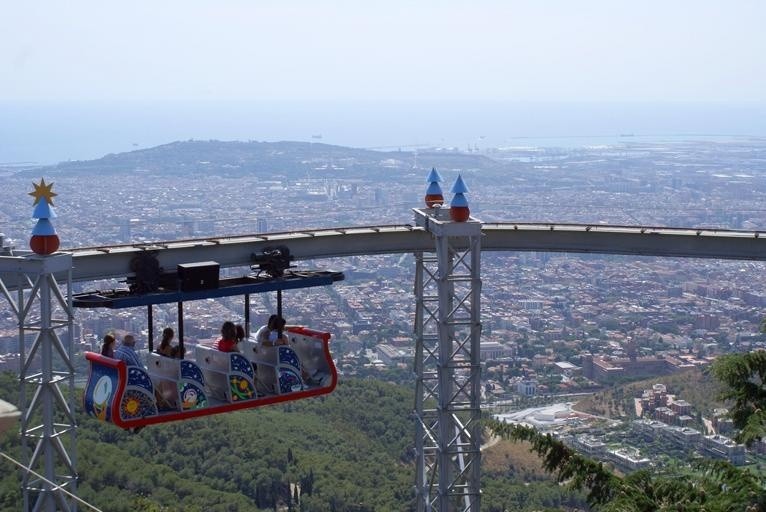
[124,334,137,344]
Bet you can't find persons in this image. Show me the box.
[100,315,320,398]
